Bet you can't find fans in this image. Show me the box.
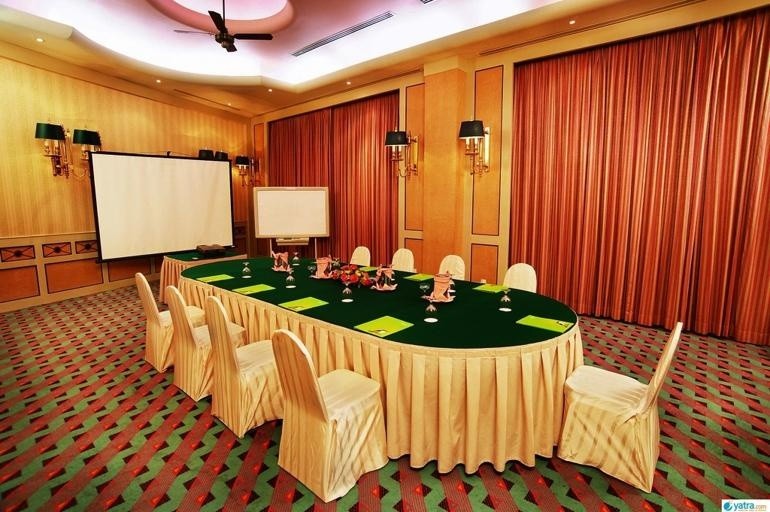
[174,1,274,54]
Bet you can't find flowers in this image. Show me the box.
[330,263,373,288]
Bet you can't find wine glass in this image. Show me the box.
[343,281,352,300]
[241,261,251,276]
[419,282,430,298]
[334,257,341,272]
[292,252,300,265]
[500,288,512,311]
[286,270,296,285]
[307,266,315,278]
[423,295,438,320]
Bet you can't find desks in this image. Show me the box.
[159,250,248,305]
[177,254,587,478]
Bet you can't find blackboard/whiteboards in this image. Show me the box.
[254,187,330,238]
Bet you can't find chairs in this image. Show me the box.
[503,262,538,294]
[266,330,388,505]
[204,293,284,440]
[557,320,685,493]
[133,272,207,377]
[164,286,249,403]
[392,248,415,273]
[439,255,466,281]
[350,246,372,267]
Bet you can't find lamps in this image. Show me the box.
[384,126,419,179]
[198,148,229,161]
[33,120,104,179]
[234,155,257,188]
[456,119,491,177]
